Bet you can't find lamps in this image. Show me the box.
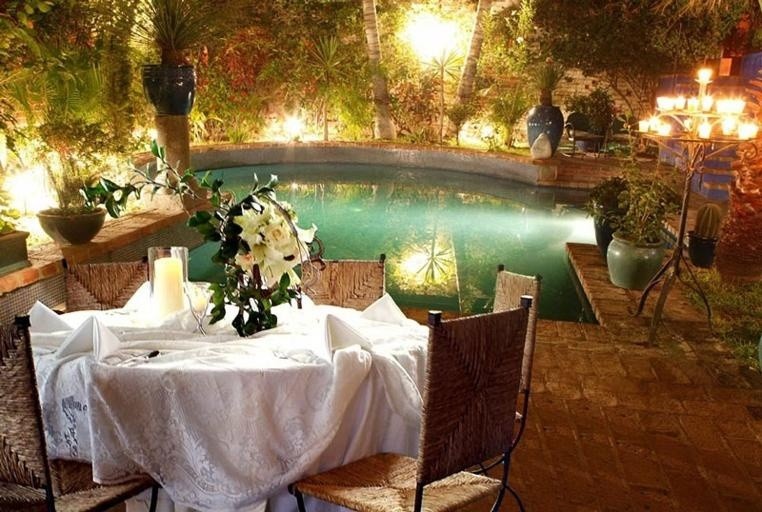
[624,66,761,348]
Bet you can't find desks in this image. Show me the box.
[32,307,429,512]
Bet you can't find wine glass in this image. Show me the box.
[182,281,215,339]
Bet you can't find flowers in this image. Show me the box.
[82,141,330,338]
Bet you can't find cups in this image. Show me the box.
[147,246,188,317]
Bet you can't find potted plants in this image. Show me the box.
[520,61,576,156]
[584,176,630,253]
[31,124,106,242]
[84,1,232,115]
[577,105,696,294]
[686,204,721,267]
[0,152,33,270]
[574,90,617,155]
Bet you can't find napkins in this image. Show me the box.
[54,315,120,360]
[300,312,372,365]
[26,299,74,333]
[121,279,154,314]
[361,293,409,328]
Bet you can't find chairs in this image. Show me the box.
[286,294,533,512]
[470,262,541,511]
[298,253,386,311]
[61,256,148,313]
[1,314,166,511]
